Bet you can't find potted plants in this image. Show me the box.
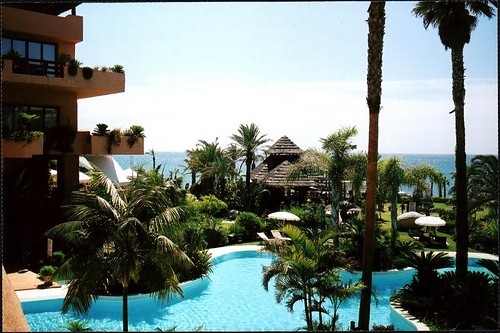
[38,266,56,283]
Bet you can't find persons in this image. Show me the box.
[399,202,406,214]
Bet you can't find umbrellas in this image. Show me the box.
[267,212,301,237]
[415,213,447,237]
[324,199,361,226]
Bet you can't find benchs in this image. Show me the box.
[412,232,433,243]
[257,231,282,247]
[269,230,292,245]
[427,236,446,247]
